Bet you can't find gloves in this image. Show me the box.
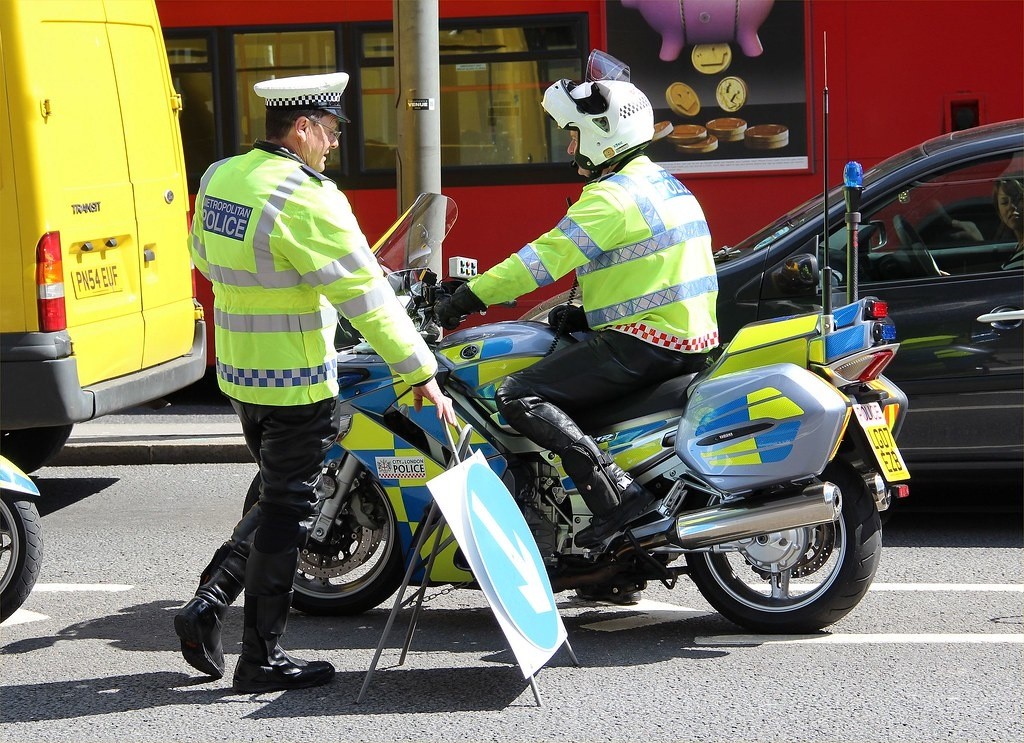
[436,284,487,330]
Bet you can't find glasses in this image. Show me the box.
[293,111,341,139]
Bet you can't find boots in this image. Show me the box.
[232,588,335,693]
[174,544,248,678]
[559,433,656,547]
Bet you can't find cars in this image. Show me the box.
[515,114,1023,512]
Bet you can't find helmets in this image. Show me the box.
[540,47,655,181]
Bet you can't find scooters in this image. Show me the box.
[1,452,44,617]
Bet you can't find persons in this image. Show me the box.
[949,171,1023,270]
[434,48,722,604]
[173,72,457,694]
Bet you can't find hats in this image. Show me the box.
[252,72,351,122]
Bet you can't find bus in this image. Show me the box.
[156,0,1023,363]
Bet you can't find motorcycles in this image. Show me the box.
[242,192,911,634]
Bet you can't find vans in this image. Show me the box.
[0,1,208,473]
[168,26,581,175]
